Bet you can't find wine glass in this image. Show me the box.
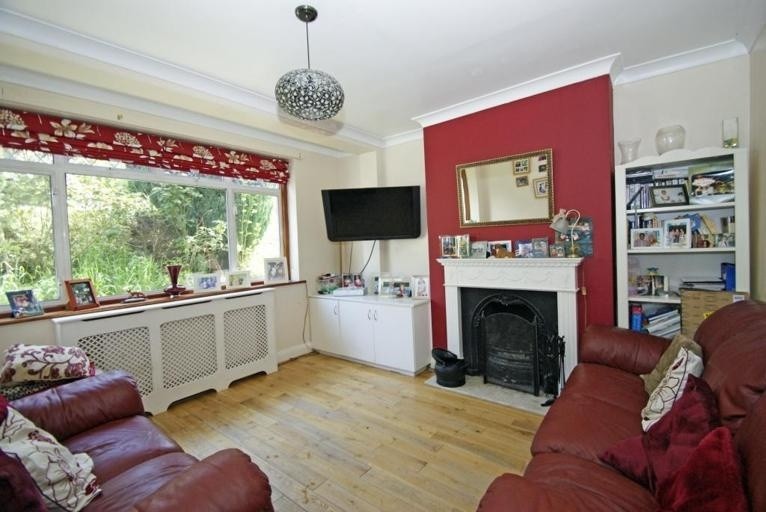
[163,264,186,294]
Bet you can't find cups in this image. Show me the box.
[722,117,740,150]
[724,264,736,291]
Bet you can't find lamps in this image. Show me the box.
[546,206,582,259]
[273,3,346,123]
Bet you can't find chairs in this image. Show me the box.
[0,366,278,512]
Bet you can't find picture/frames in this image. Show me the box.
[263,256,289,287]
[62,277,100,312]
[193,273,220,291]
[410,272,431,301]
[437,233,514,258]
[662,217,693,249]
[227,270,252,290]
[510,155,548,200]
[648,181,691,209]
[629,225,663,248]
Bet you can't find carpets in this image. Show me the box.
[423,370,554,417]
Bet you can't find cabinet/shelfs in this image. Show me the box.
[304,290,432,378]
[613,143,754,330]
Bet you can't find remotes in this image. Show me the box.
[124,297,144,303]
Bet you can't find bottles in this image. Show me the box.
[617,136,641,164]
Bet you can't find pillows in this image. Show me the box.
[595,322,753,511]
[0,392,104,512]
[0,339,90,388]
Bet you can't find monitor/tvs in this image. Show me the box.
[321,185,421,242]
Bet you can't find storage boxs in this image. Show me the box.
[680,289,734,341]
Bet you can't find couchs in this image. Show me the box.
[472,294,766,512]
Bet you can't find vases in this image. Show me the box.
[720,115,741,150]
[617,136,644,165]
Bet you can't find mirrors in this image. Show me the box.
[451,145,560,231]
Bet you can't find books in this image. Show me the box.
[626,166,735,341]
